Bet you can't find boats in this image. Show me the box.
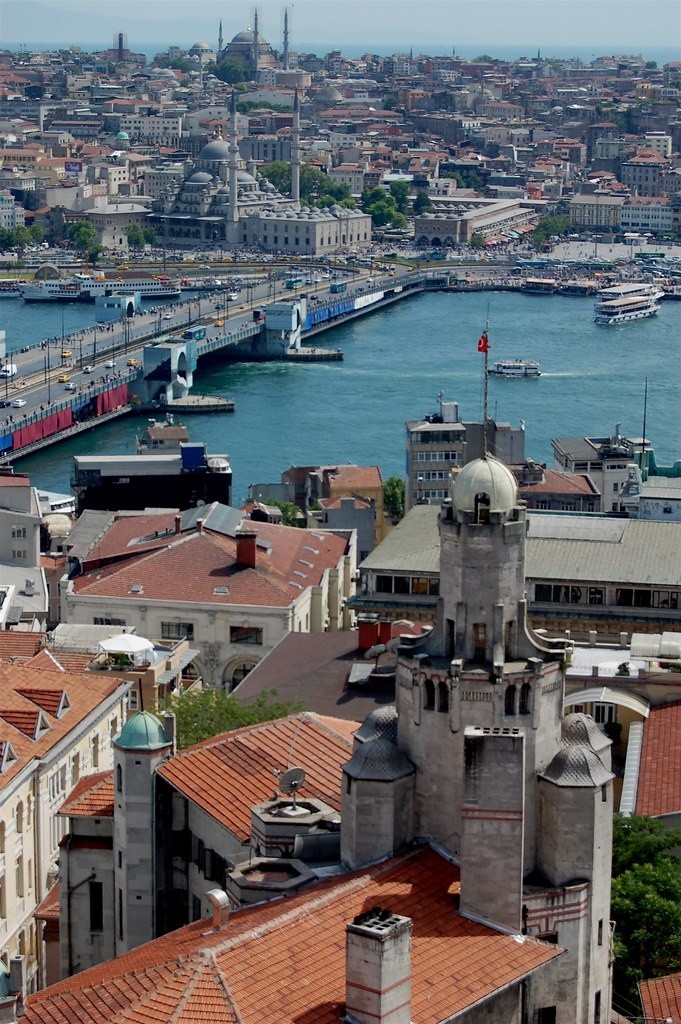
[489,357,541,380]
[0,290,22,298]
[521,278,556,295]
[558,283,591,297]
[592,296,662,327]
[597,283,665,305]
[15,253,182,302]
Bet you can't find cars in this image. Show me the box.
[215,303,224,310]
[311,295,318,300]
[0,351,115,409]
[214,320,224,327]
[233,249,414,306]
[501,253,681,286]
[164,312,175,320]
[126,358,138,367]
[227,294,238,301]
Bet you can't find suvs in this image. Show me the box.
[198,264,210,270]
[299,294,308,299]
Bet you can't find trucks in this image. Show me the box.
[253,309,265,323]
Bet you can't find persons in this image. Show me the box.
[366,239,594,259]
[205,322,248,345]
[78,370,122,395]
[470,273,617,290]
[22,403,55,419]
[120,291,215,318]
[6,414,12,426]
[307,284,378,308]
[51,323,109,343]
[21,339,47,353]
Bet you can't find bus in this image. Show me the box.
[285,278,303,289]
[330,281,347,293]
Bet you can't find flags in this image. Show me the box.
[477,333,491,353]
